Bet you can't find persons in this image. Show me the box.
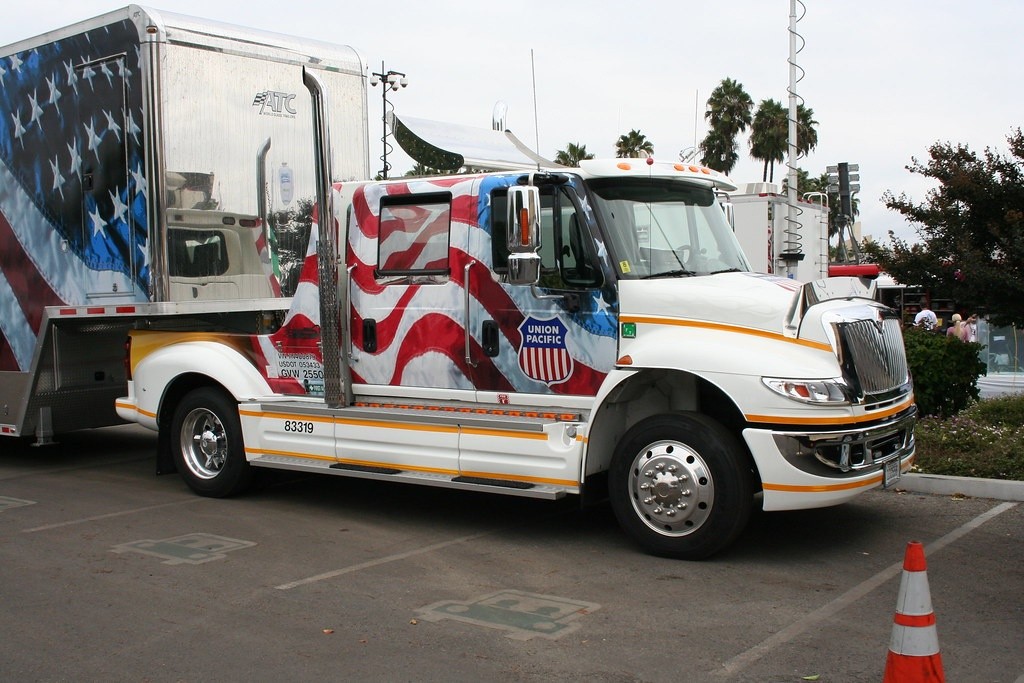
[913,298,938,331]
[945,314,978,342]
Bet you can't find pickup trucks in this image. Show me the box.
[114,159,917,561]
[166,208,282,300]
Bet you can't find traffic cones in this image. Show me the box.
[883,541,946,683]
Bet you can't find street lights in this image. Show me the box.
[370,60,408,180]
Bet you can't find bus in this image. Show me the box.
[0,4,374,446]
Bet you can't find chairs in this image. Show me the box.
[569,210,660,280]
